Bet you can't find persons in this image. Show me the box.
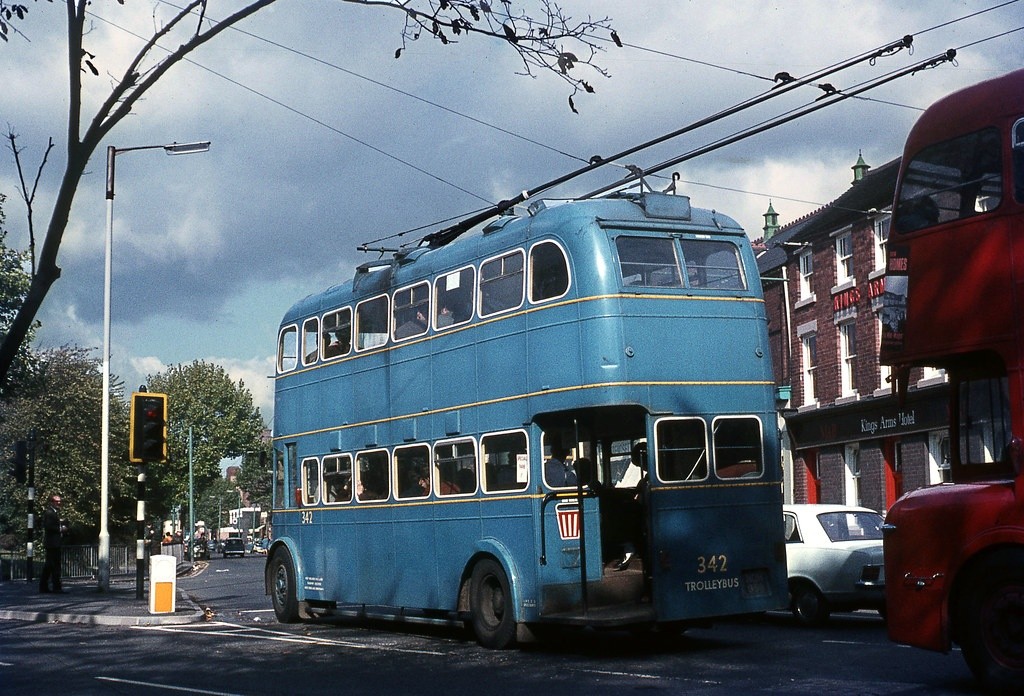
[306,327,363,363]
[485,446,527,491]
[617,443,648,567]
[197,533,207,545]
[39,495,70,594]
[416,303,451,321]
[400,462,475,498]
[166,532,172,543]
[314,466,337,503]
[546,441,601,496]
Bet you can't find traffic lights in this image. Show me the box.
[129,393,168,463]
[9,441,28,483]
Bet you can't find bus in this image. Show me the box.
[264,34,959,650]
[878,66,1024,695]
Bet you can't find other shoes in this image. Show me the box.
[39,590,53,593]
[53,590,70,594]
[618,553,636,566]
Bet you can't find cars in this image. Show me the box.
[184,540,225,561]
[223,537,245,559]
[783,504,886,628]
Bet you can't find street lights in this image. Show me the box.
[96,142,212,599]
[209,495,222,541]
[227,489,241,538]
[252,503,257,538]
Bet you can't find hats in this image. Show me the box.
[195,520,204,526]
[550,439,570,454]
